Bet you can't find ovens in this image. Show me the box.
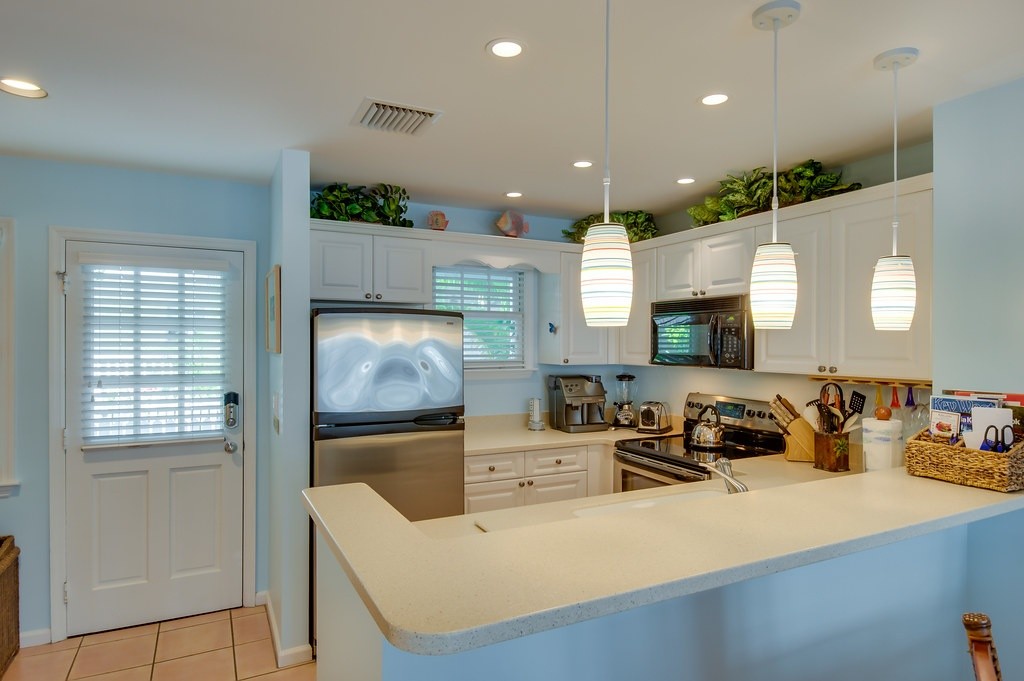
[612,441,711,495]
[649,292,754,372]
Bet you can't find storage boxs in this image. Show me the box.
[905,423,1024,493]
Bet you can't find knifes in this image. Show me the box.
[768,393,801,438]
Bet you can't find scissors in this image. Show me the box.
[985,425,1014,453]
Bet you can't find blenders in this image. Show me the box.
[610,374,639,427]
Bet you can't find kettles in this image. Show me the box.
[688,403,726,450]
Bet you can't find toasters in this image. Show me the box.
[636,400,673,434]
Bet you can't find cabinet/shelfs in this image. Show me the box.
[310,217,440,304]
[615,252,656,365]
[655,226,754,304]
[559,241,621,366]
[753,169,930,380]
[463,442,590,514]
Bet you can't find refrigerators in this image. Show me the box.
[309,310,466,657]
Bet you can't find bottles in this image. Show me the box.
[951,613,1003,680]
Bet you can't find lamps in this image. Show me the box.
[579,1,635,329]
[753,1,800,330]
[871,46,916,331]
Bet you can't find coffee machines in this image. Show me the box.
[546,373,609,433]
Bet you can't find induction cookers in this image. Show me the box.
[614,392,793,480]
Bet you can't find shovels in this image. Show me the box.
[842,390,866,427]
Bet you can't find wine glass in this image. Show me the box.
[861,383,931,439]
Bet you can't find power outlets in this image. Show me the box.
[272,416,279,433]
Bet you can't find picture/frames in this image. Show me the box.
[265,264,281,355]
